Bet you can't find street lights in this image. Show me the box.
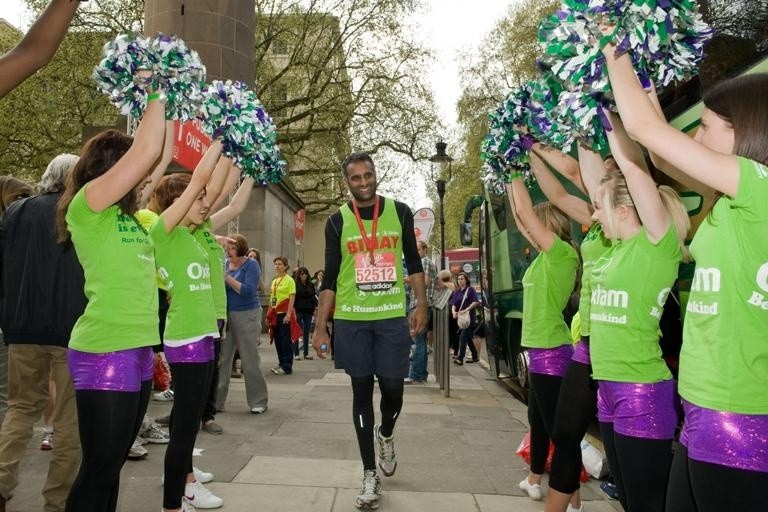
[425,134,454,273]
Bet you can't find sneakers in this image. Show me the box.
[41,430,55,450]
[201,422,224,434]
[295,355,300,360]
[139,423,171,443]
[270,366,286,375]
[306,355,313,360]
[518,471,550,503]
[375,424,397,475]
[467,359,479,364]
[153,388,175,401]
[129,441,147,457]
[250,406,265,412]
[455,359,463,365]
[155,415,171,426]
[162,467,223,512]
[355,469,381,509]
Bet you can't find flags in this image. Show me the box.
[412,207,435,244]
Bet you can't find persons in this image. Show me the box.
[264,257,334,375]
[1,70,268,511]
[311,154,429,510]
[402,241,483,385]
[505,15,768,511]
[1,1,82,98]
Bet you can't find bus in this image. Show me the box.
[441,246,481,290]
[459,1,767,399]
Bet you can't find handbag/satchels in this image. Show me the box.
[457,310,471,328]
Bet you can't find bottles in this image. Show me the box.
[318,343,332,360]
